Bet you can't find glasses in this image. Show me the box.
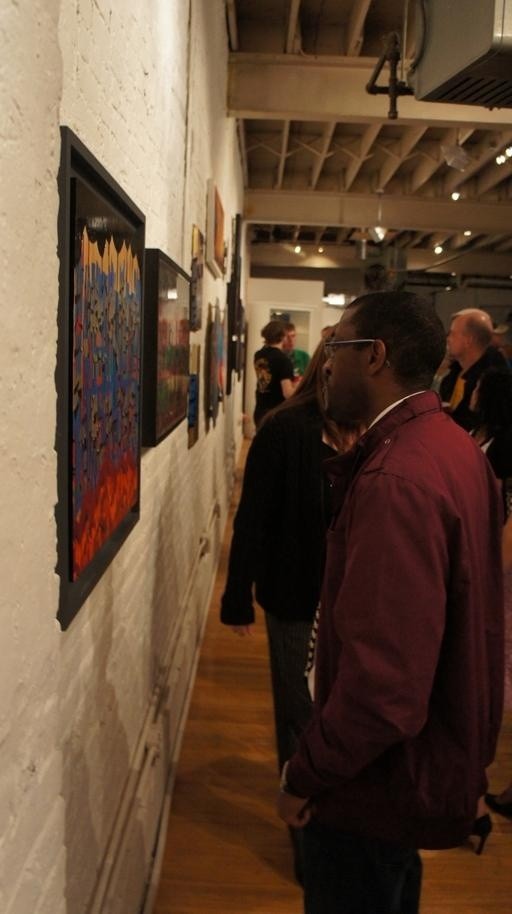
[324,332,391,369]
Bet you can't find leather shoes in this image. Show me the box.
[485,793,512,818]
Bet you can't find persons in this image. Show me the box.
[320,324,331,340]
[274,287,508,913]
[281,321,312,374]
[220,339,369,889]
[430,307,511,544]
[253,320,301,427]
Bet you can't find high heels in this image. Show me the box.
[468,814,491,854]
